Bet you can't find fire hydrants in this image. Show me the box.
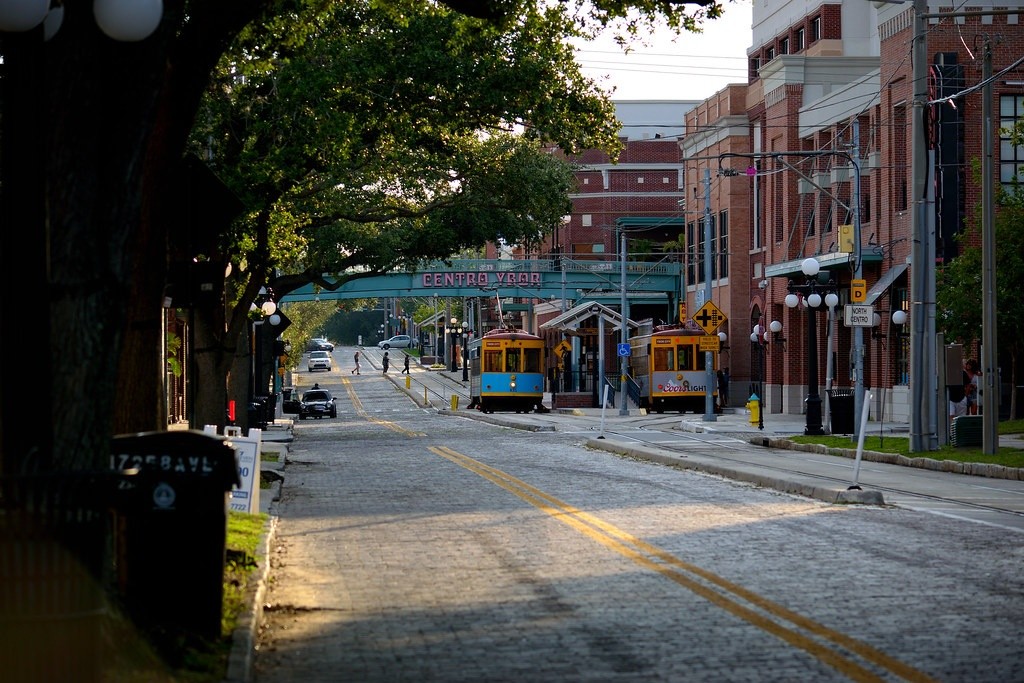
[745,393,760,427]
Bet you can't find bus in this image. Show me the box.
[628,323,719,414]
[469,328,546,414]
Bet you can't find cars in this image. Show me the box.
[307,338,334,352]
[300,388,337,420]
[307,351,332,373]
[378,335,419,350]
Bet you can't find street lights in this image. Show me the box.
[462,322,475,381]
[784,258,839,435]
[554,214,572,271]
[248,286,277,400]
[445,318,462,372]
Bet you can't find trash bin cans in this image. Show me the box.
[282,389,293,400]
[110,433,241,642]
[825,389,855,434]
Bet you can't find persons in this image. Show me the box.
[948,368,971,422]
[383,352,389,373]
[402,354,410,374]
[312,383,320,389]
[718,365,729,407]
[352,351,360,375]
[964,359,978,415]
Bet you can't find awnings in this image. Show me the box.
[862,263,909,308]
